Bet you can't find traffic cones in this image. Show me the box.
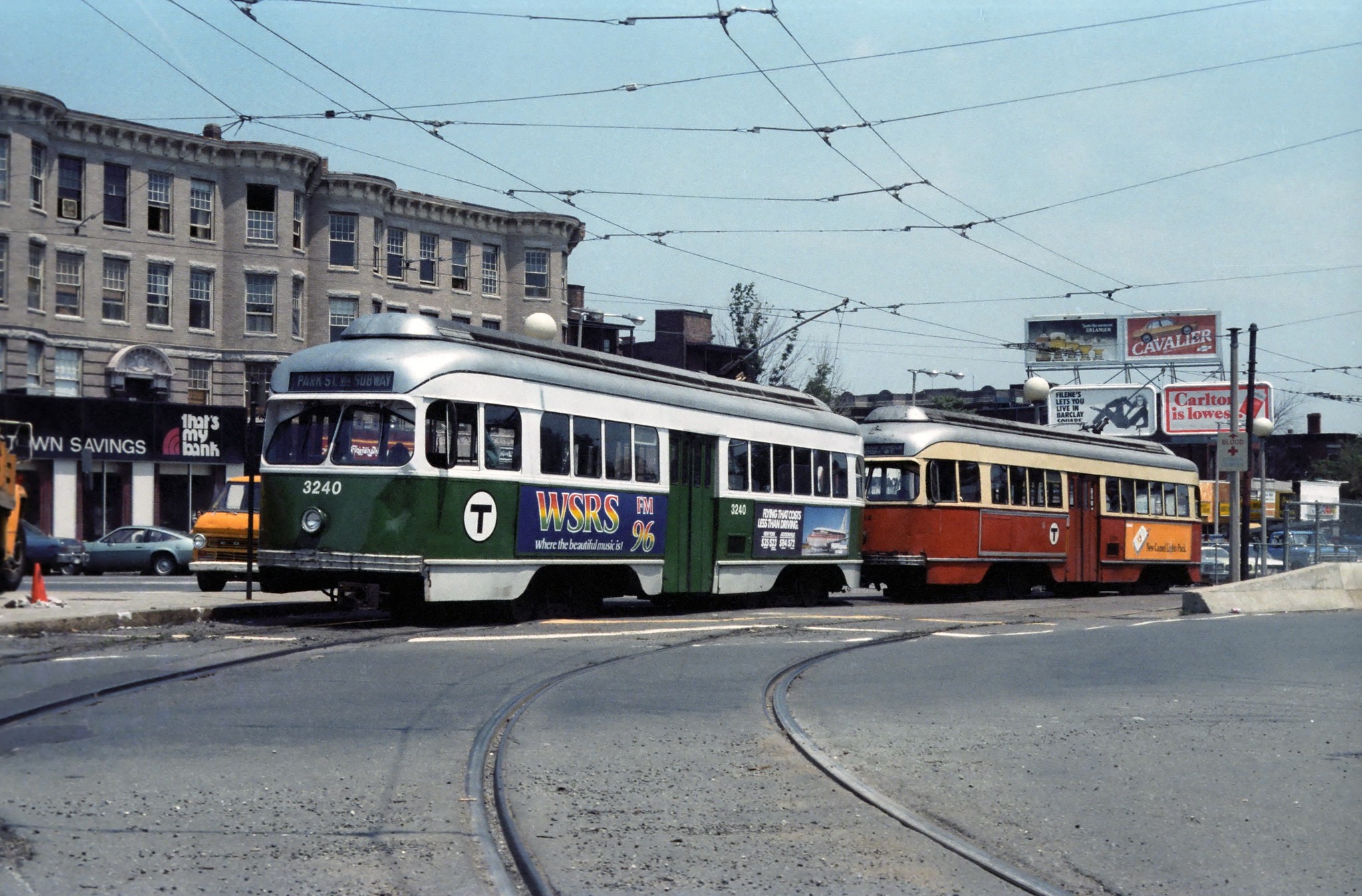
[31,565,51,604]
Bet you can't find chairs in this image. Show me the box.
[388,443,409,465]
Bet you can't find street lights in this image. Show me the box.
[1252,416,1275,577]
[1024,376,1050,424]
[909,368,965,407]
[570,307,645,350]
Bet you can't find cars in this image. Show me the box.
[1269,531,1358,571]
[1198,544,1284,581]
[60,526,195,575]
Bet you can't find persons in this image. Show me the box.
[1224,536,1229,542]
[1082,394,1149,430]
[1204,535,1211,541]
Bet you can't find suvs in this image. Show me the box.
[188,475,262,592]
[0,420,89,594]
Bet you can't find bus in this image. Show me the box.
[246,311,866,625]
[858,405,1201,603]
